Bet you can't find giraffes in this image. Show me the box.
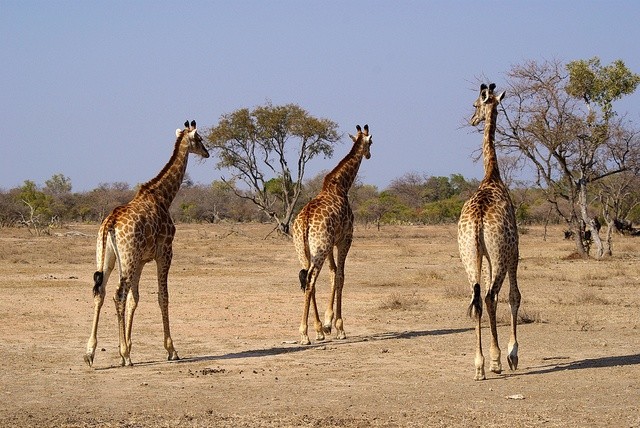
[456,83,521,381]
[292,124,373,345]
[83,119,210,368]
